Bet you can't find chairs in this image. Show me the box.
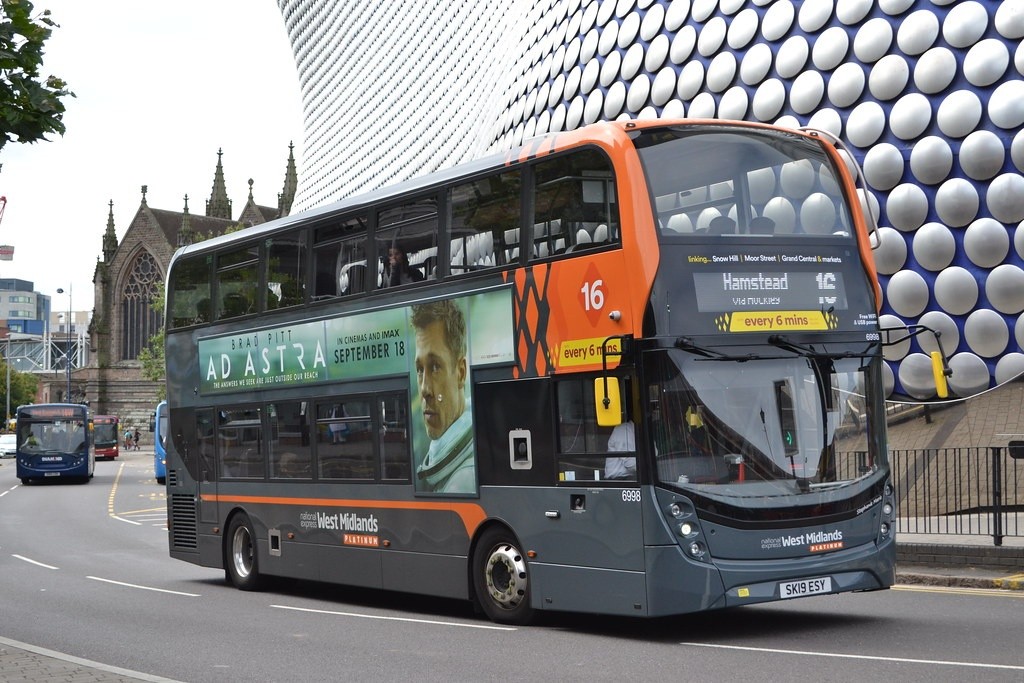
[196,256,439,324]
[510,217,775,266]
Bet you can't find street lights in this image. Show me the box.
[56,283,73,403]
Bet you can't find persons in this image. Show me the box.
[134,428,141,450]
[604,395,658,480]
[413,299,476,495]
[21,430,43,447]
[384,245,423,288]
[125,429,132,450]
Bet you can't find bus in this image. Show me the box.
[77,414,123,461]
[9,401,96,485]
[149,401,168,484]
[161,116,955,627]
